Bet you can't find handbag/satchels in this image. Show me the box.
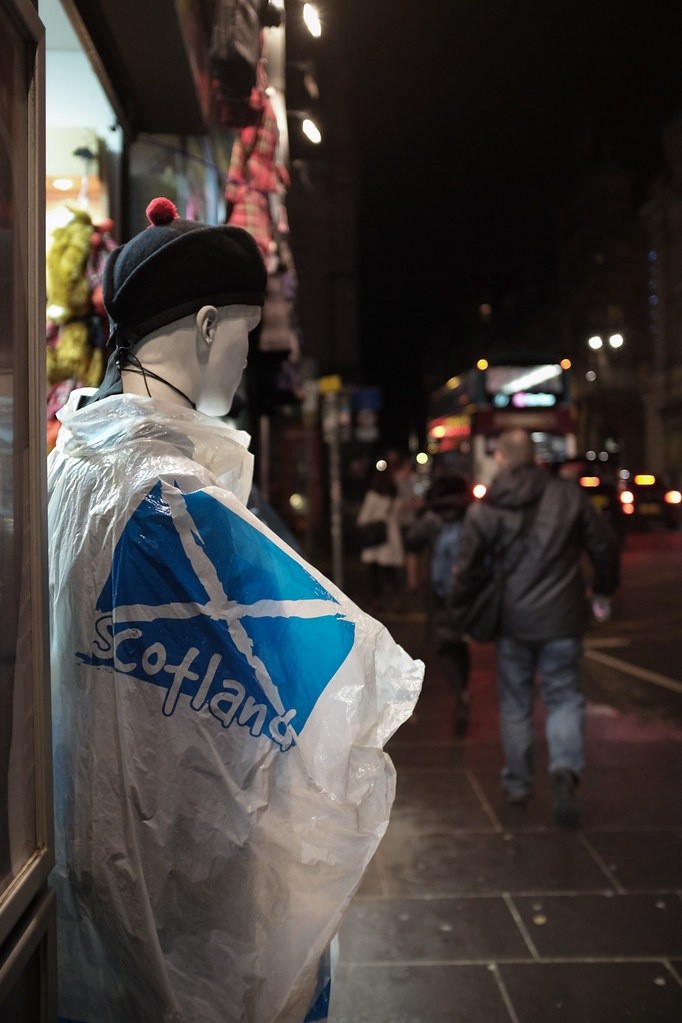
[462,582,503,643]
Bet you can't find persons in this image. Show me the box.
[454,428,621,822]
[361,456,482,736]
[48,198,427,1022]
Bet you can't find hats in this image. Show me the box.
[427,475,464,507]
[101,197,267,366]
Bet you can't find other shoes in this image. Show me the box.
[503,784,529,804]
[551,768,581,826]
[451,705,469,738]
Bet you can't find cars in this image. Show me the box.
[616,470,682,531]
[577,479,626,531]
[426,473,482,521]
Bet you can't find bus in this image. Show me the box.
[425,361,581,483]
